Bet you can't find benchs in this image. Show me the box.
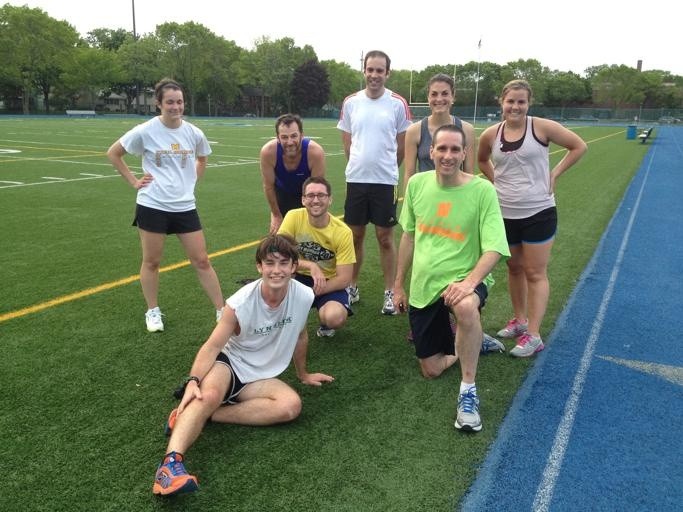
[637,128,652,144]
[643,127,652,135]
[66,111,96,118]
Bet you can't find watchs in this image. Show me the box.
[183,376,201,390]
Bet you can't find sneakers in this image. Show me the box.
[496,318,529,339]
[381,289,399,316]
[407,327,414,342]
[346,285,360,305]
[145,305,165,333]
[317,325,337,338]
[479,330,507,354]
[454,389,484,433]
[215,307,225,323]
[151,452,201,497]
[164,408,177,438]
[509,331,545,358]
[448,311,458,335]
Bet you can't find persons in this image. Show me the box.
[152,234,335,495]
[106,78,227,334]
[337,50,412,317]
[477,80,588,359]
[276,176,357,338]
[260,113,326,236]
[404,72,475,342]
[393,124,511,431]
